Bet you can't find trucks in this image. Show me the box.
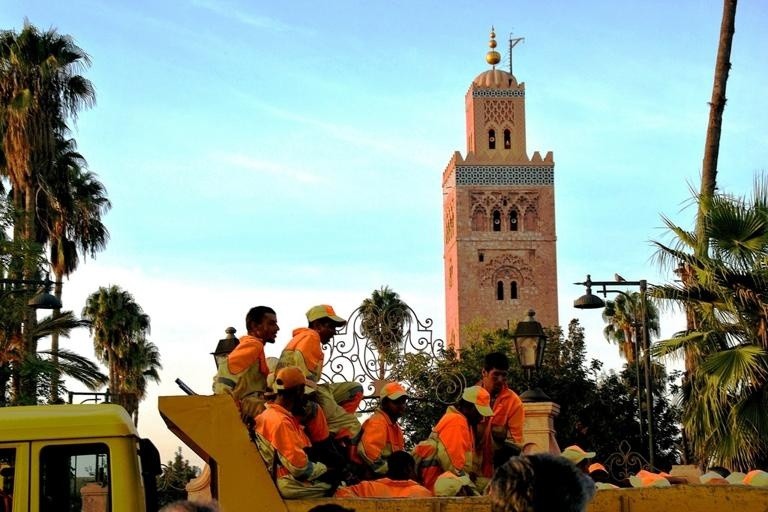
[0,394,768,512]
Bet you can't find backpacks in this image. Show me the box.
[412,439,442,497]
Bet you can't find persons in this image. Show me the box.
[212,306,279,425]
[629,465,768,486]
[560,445,618,489]
[419,386,495,477]
[490,452,596,512]
[332,450,432,498]
[274,304,363,464]
[253,366,331,499]
[474,351,524,471]
[435,470,480,496]
[357,382,410,475]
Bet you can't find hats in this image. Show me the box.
[433,471,470,497]
[275,365,317,391]
[630,469,670,487]
[594,482,620,488]
[462,385,496,419]
[379,383,413,404]
[561,445,597,465]
[701,469,747,487]
[306,306,347,325]
[745,469,768,488]
[587,463,608,475]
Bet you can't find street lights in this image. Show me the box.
[0,272,63,309]
[572,274,656,472]
[512,308,547,389]
[210,326,240,369]
